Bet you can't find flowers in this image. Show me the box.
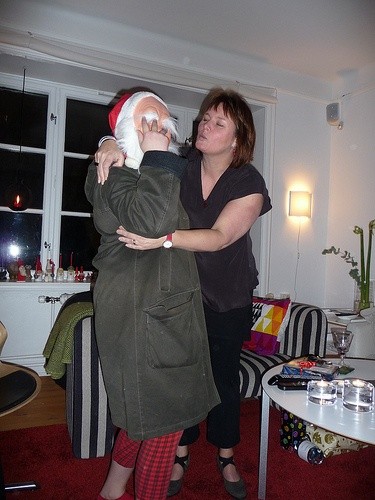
[322,219,375,283]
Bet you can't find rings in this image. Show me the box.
[133,239,135,245]
[94,162,99,166]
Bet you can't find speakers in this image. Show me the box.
[326,103,340,123]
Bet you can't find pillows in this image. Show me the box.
[243,296,292,356]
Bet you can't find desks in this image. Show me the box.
[257,359,375,500]
[324,310,375,358]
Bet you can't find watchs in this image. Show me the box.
[162,234,173,249]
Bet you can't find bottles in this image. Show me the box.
[17,255,85,282]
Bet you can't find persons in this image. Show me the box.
[95,87,272,499]
[84,91,223,500]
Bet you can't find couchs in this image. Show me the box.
[65,291,328,460]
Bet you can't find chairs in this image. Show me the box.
[0,322,40,500]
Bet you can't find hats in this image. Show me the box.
[108,91,169,169]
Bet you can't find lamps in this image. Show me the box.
[288,190,312,218]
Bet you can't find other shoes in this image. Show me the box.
[97,490,135,500]
[167,454,189,496]
[217,455,247,500]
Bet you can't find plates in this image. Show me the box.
[334,312,359,321]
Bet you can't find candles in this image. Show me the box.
[15,196,22,208]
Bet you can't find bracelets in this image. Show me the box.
[98,136,116,148]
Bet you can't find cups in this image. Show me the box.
[307,380,335,406]
[342,378,375,413]
[330,380,346,398]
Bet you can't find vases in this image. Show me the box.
[352,281,375,320]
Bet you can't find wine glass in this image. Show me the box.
[330,328,354,367]
